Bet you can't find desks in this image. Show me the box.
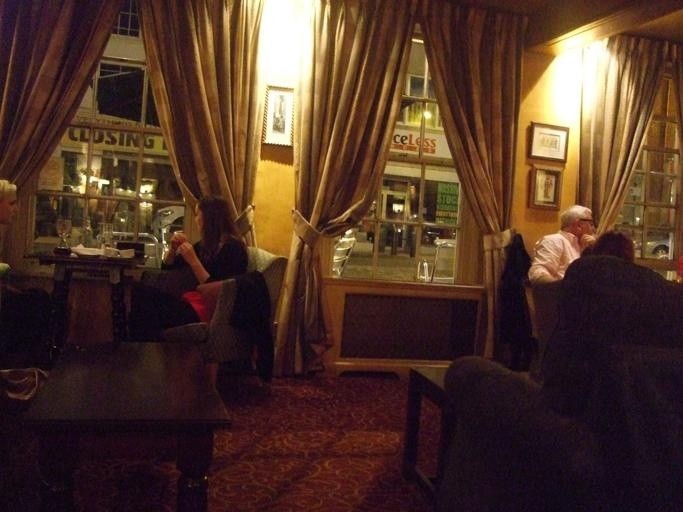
[19,340,232,511]
[400,365,534,502]
[24,250,150,348]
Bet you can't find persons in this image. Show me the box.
[527,202,599,284]
[127,195,247,345]
[582,228,637,262]
[0,180,54,370]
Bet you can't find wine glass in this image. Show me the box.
[56,219,72,249]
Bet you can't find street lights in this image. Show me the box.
[412,107,433,266]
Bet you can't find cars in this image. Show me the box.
[366,213,454,247]
[155,203,185,235]
[631,236,671,257]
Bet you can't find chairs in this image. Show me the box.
[483,227,543,359]
[438,254,682,511]
[140,246,288,405]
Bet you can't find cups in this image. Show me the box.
[105,246,135,259]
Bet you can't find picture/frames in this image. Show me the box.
[528,121,570,210]
[261,86,295,148]
